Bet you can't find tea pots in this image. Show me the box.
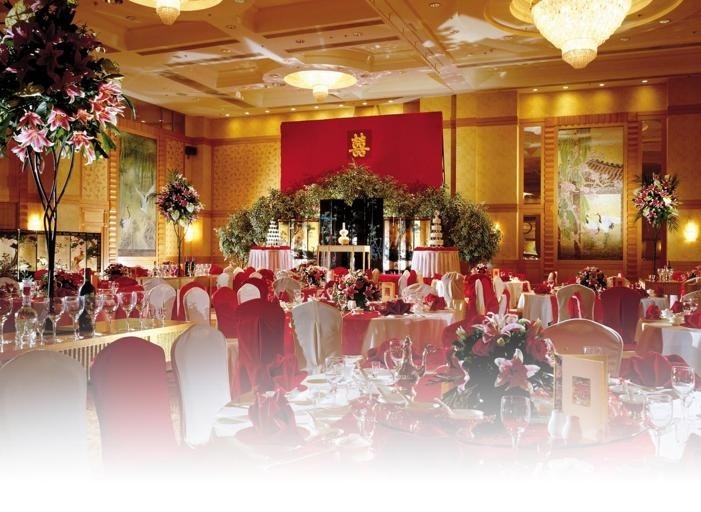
[385,334,432,383]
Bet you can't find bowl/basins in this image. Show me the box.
[609,378,650,414]
[448,408,486,430]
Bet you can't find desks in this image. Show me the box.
[249,245,292,269]
[412,246,459,276]
[317,244,370,274]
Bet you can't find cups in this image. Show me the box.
[349,300,357,312]
[683,297,699,315]
[649,274,656,282]
[548,411,572,439]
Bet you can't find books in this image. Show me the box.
[552,352,610,444]
[381,282,396,303]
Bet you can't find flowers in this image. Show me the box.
[634,174,682,274]
[1,0,136,335]
[158,170,206,280]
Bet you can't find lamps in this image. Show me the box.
[126,0,224,26]
[274,62,359,99]
[530,1,631,72]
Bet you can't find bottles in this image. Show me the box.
[16,280,38,340]
[667,260,672,272]
[78,266,97,330]
[185,255,196,276]
[332,283,339,301]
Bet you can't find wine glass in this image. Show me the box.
[0,289,170,344]
[306,340,415,451]
[146,263,212,278]
[508,272,513,280]
[644,394,674,452]
[294,287,305,305]
[549,280,555,293]
[657,268,674,282]
[327,287,348,314]
[672,362,695,420]
[498,395,532,452]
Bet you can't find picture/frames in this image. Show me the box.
[115,124,160,262]
[551,124,629,265]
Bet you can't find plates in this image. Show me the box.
[235,424,311,451]
